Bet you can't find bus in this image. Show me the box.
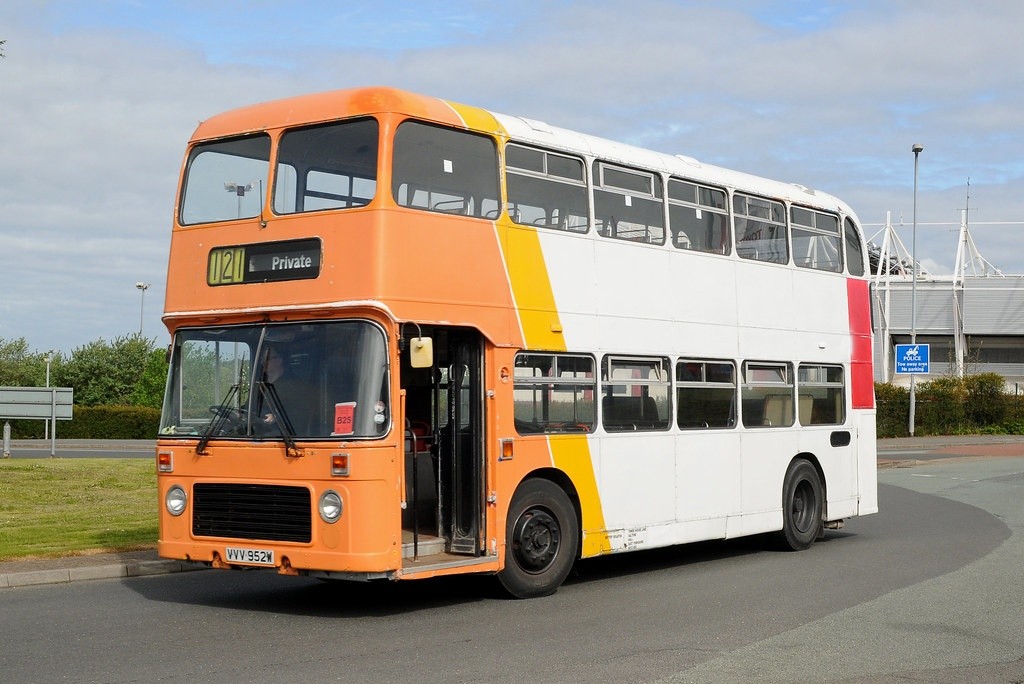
[156,86,877,600]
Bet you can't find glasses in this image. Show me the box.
[262,356,278,361]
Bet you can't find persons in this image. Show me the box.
[232,342,313,436]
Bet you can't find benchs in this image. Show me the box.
[603,396,660,428]
[437,201,840,273]
[762,394,812,425]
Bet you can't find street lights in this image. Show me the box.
[224,182,252,409]
[909,143,924,437]
[136,282,152,339]
